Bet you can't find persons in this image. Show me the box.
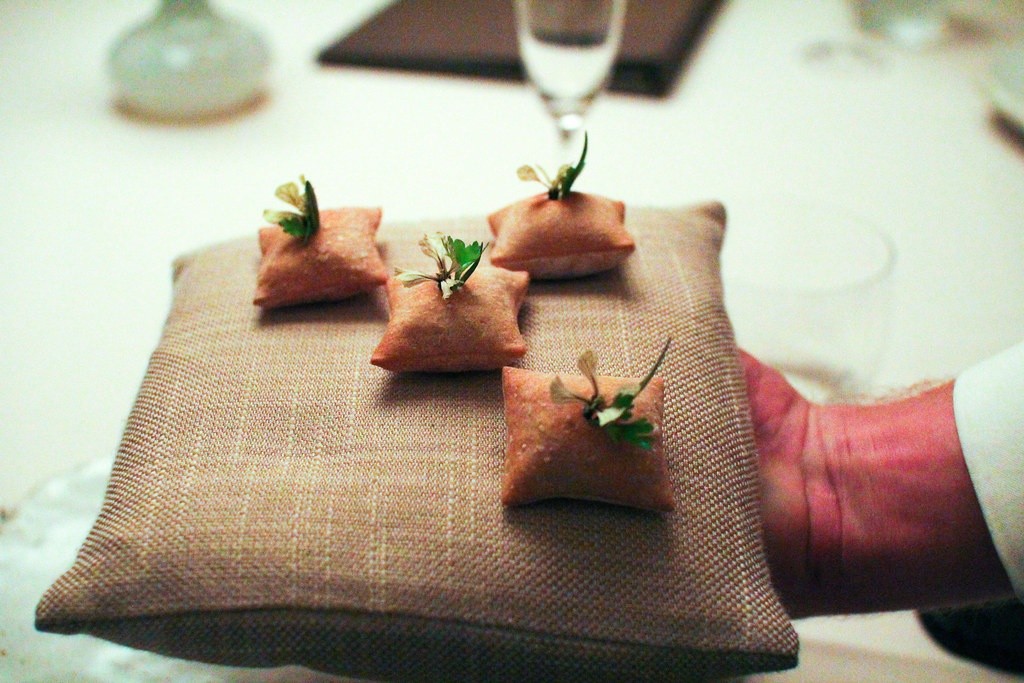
[738,340,1024,621]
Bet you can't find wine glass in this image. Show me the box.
[514,0,625,165]
